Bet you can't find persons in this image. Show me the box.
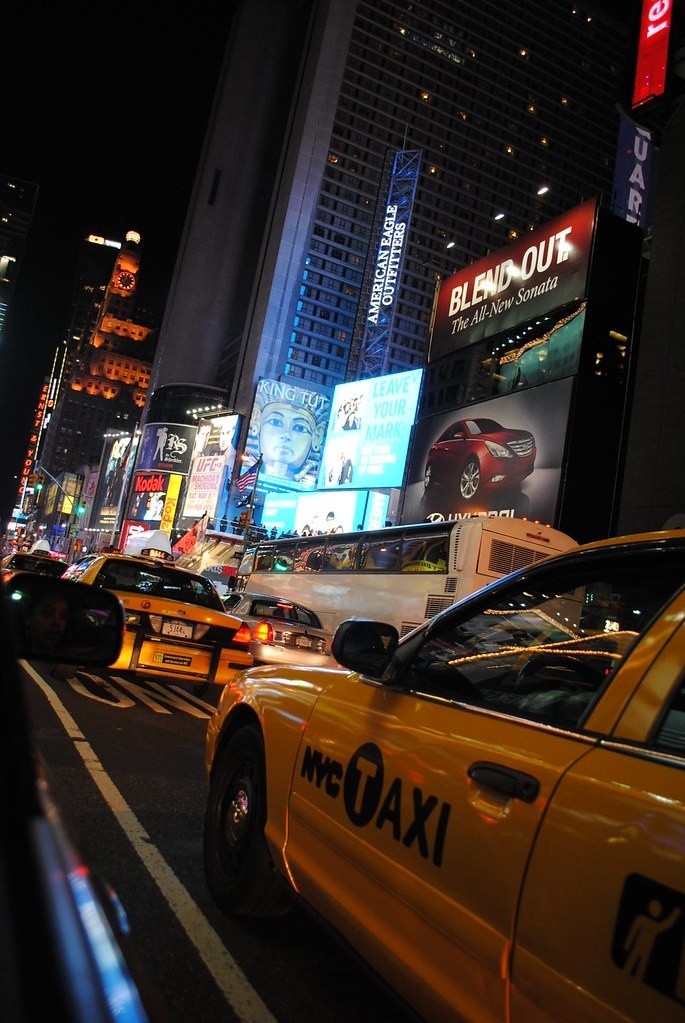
[325,451,353,485]
[21,591,70,654]
[302,511,343,536]
[335,394,363,431]
[192,416,236,461]
[202,510,298,541]
[104,439,122,482]
[131,492,163,520]
[246,378,330,485]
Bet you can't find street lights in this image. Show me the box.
[242,452,262,535]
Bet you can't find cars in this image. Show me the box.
[60,530,253,692]
[201,524,684,1021]
[0,539,71,586]
[219,593,333,668]
[426,420,537,501]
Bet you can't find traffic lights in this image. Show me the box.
[37,474,45,491]
[14,529,20,536]
[72,543,78,552]
[28,474,38,488]
[79,544,87,553]
[78,499,86,513]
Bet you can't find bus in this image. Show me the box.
[227,516,582,642]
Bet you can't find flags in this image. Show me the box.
[237,492,252,508]
[235,462,259,492]
